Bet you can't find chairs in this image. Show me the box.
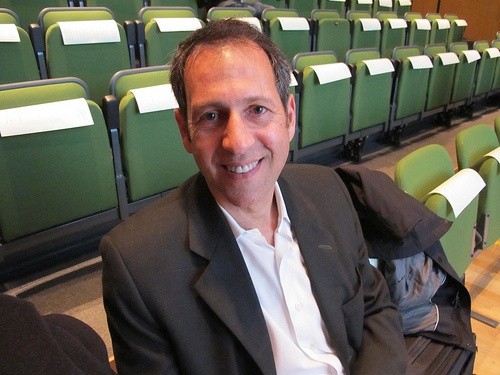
[0,0,500,375]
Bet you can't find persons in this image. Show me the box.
[99,19,408,375]
[0,293,115,375]
